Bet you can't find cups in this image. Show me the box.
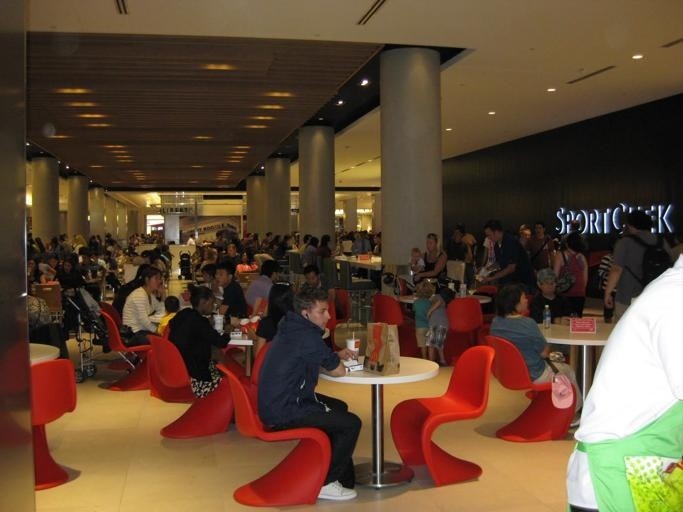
[460,284,467,298]
[351,256,356,262]
[214,315,223,333]
[448,283,455,292]
[346,338,360,360]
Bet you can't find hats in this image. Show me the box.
[536,268,556,283]
[261,260,283,269]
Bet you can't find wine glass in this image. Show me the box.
[368,251,373,264]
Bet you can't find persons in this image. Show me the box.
[257,285,363,501]
[26,209,675,399]
[488,283,585,427]
[564,251,683,512]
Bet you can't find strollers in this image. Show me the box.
[63,287,143,383]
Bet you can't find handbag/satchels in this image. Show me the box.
[380,272,400,297]
[550,371,573,409]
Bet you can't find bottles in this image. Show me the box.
[543,305,551,329]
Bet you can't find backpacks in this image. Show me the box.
[619,233,673,287]
[555,271,576,295]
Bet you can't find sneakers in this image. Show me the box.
[317,481,358,502]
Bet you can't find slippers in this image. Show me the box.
[434,360,448,366]
